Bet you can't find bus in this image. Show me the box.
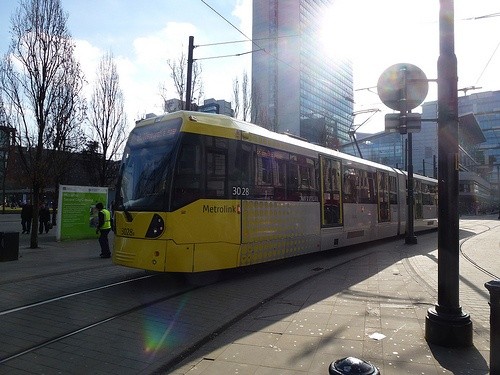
[111,110,438,273]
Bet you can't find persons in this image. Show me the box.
[21,200,57,235]
[95,202,112,259]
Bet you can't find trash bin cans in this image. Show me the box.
[1,231,20,261]
